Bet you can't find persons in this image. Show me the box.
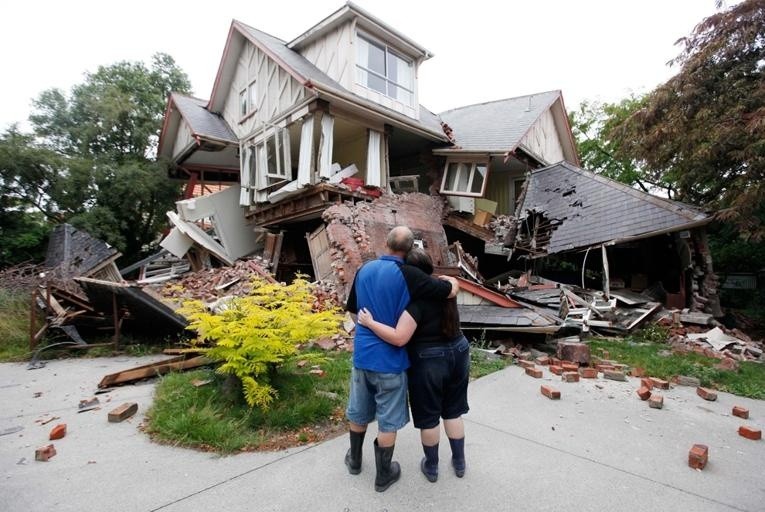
[358,247,471,481]
[342,227,460,493]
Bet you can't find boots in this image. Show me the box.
[343,430,366,475]
[373,437,401,492]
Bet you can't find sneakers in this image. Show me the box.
[420,456,438,483]
[451,459,466,478]
